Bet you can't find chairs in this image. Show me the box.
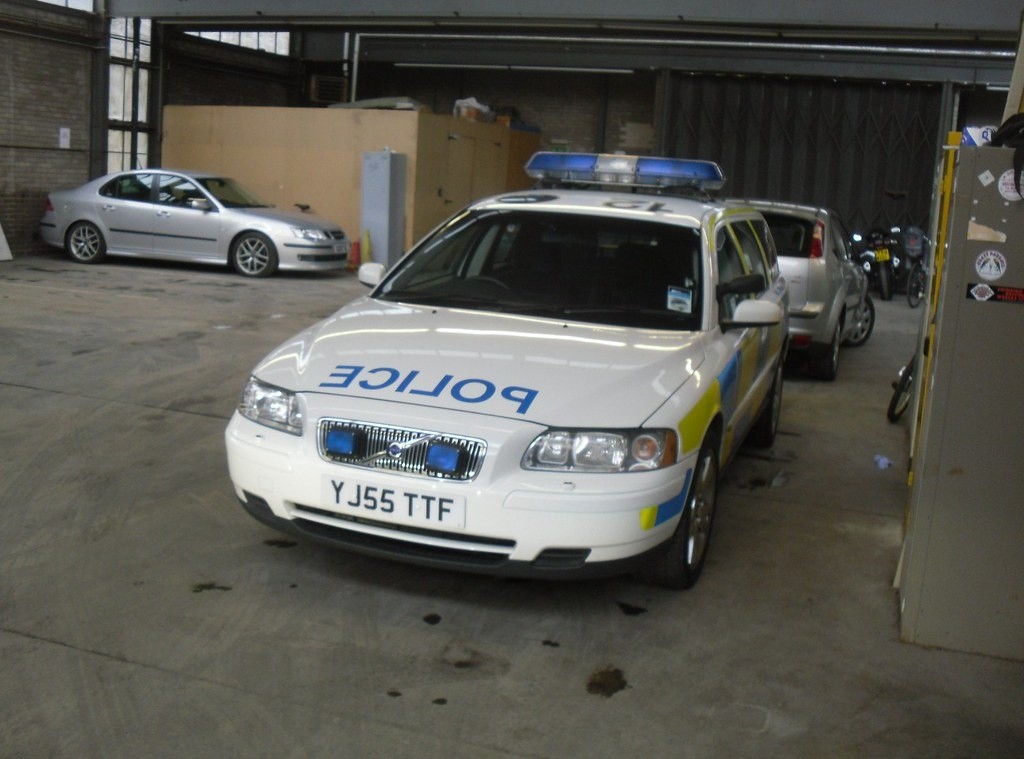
[494,226,573,301]
[589,241,679,307]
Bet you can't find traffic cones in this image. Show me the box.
[356,229,374,268]
[345,237,360,272]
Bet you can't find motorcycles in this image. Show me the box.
[850,224,900,301]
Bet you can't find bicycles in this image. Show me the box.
[887,353,916,422]
[906,233,947,308]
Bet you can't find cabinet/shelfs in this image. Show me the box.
[898,143,1023,665]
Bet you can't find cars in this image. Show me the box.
[717,194,876,382]
[225,152,793,593]
[38,165,353,278]
